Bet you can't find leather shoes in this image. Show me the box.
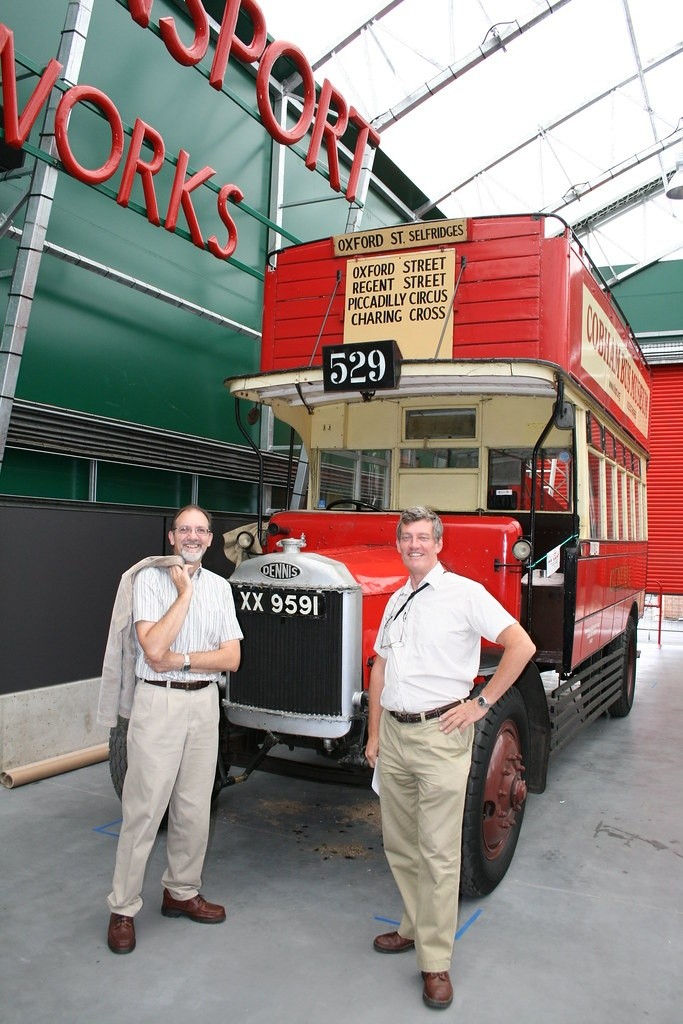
[373,930,415,954]
[421,971,453,1008]
[161,888,226,924]
[108,913,136,954]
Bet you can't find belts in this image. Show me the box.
[390,698,468,723]
[140,678,212,692]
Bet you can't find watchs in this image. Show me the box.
[477,692,492,708]
[181,653,191,673]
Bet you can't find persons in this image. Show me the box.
[107,504,245,956]
[364,506,536,1009]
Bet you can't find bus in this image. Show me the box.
[109,210,653,902]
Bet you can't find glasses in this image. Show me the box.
[172,526,213,536]
[380,612,407,652]
